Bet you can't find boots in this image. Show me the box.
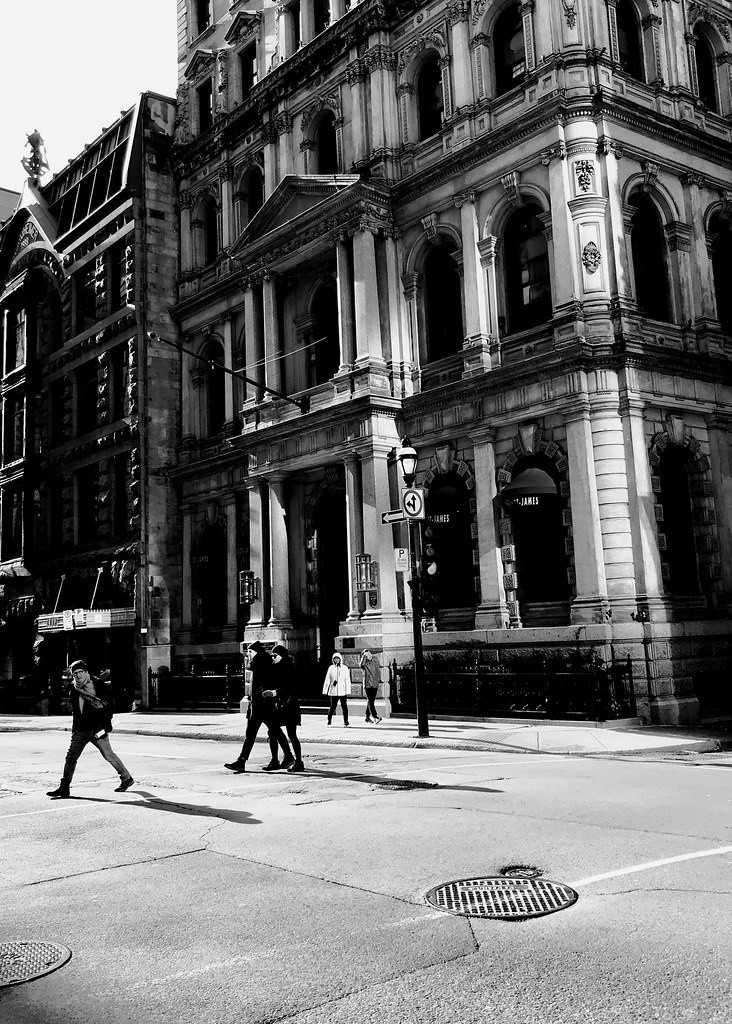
[47,778,70,797]
[115,776,134,791]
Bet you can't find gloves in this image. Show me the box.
[262,690,273,697]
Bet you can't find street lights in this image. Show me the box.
[397,434,429,738]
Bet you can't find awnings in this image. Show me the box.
[36,606,137,633]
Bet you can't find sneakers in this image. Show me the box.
[280,753,295,769]
[287,760,305,772]
[262,758,280,771]
[224,758,246,772]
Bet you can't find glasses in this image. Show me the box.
[74,671,83,676]
[270,654,278,658]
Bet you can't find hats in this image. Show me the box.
[272,645,288,657]
[248,641,262,652]
[69,660,86,675]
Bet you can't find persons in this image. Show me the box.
[321,651,354,729]
[223,640,295,771]
[261,644,305,773]
[358,648,382,724]
[46,659,134,799]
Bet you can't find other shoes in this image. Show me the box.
[366,718,374,723]
[374,717,382,724]
